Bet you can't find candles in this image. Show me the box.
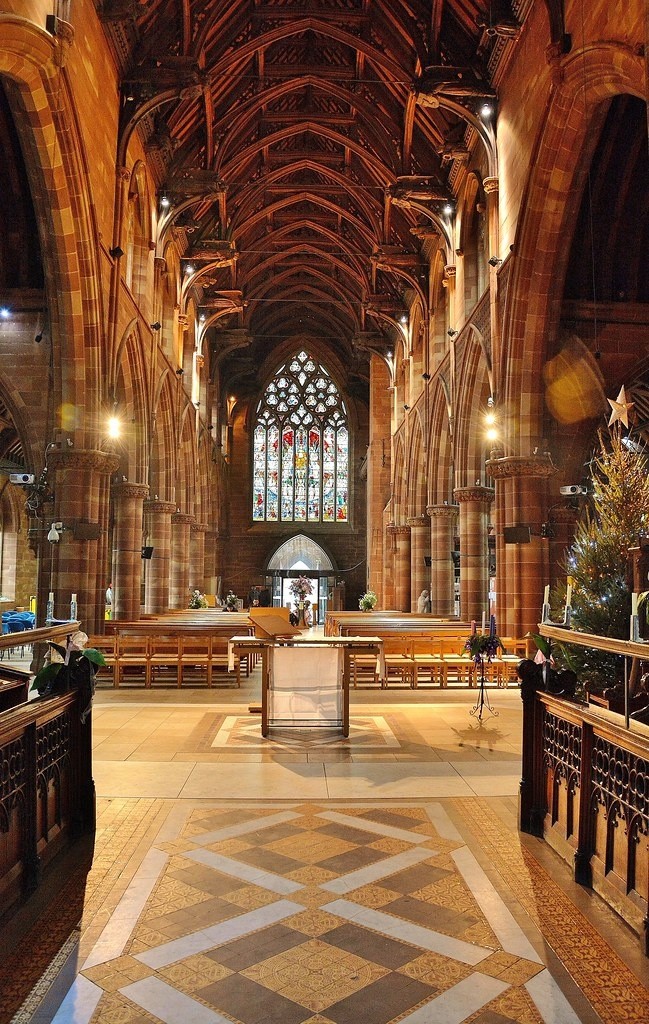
[567,583,572,606]
[471,611,496,637]
[544,584,550,604]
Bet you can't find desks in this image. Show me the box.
[229,636,384,738]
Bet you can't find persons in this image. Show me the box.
[223,602,239,612]
[106,583,112,605]
[417,589,431,613]
[246,598,263,612]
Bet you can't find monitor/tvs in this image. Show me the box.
[141,547,153,559]
[451,551,460,563]
[425,557,431,566]
[74,522,100,540]
[503,527,531,544]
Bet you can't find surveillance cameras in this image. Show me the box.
[47,529,59,544]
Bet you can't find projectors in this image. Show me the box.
[559,485,587,495]
[9,473,35,484]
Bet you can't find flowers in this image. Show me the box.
[188,590,208,609]
[358,586,378,613]
[462,633,507,663]
[289,575,313,597]
[227,591,238,604]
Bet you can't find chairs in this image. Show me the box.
[0,610,35,661]
[353,637,527,689]
[583,662,649,725]
[85,634,242,689]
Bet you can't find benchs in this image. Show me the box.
[324,610,533,671]
[84,607,262,681]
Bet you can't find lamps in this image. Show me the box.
[151,321,161,331]
[488,255,503,267]
[446,328,459,338]
[110,246,124,260]
[422,373,430,381]
[176,368,183,375]
[35,330,44,343]
[403,404,410,411]
